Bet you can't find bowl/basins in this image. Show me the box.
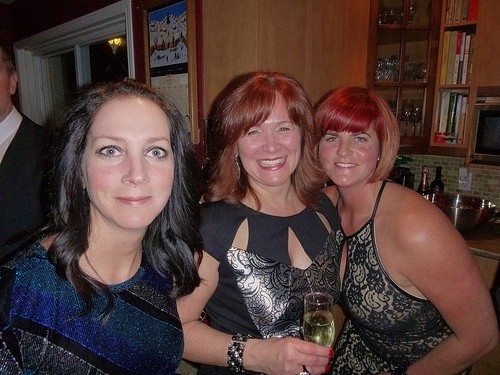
[428,193,497,232]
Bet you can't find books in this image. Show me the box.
[438,0,479,84]
[432,87,470,145]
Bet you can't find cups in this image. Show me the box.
[302,293,335,349]
[375,55,427,83]
[379,0,416,24]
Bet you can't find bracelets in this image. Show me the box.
[227,332,254,374]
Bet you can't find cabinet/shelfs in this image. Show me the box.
[365,0,500,171]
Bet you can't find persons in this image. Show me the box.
[0,77,205,375]
[0,43,56,253]
[313,84,500,375]
[176,71,345,375]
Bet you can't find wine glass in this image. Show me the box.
[387,99,423,130]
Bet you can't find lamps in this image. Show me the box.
[107,36,123,54]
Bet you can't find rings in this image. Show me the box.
[299,365,312,375]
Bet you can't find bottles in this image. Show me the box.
[430,166,445,199]
[416,164,430,201]
